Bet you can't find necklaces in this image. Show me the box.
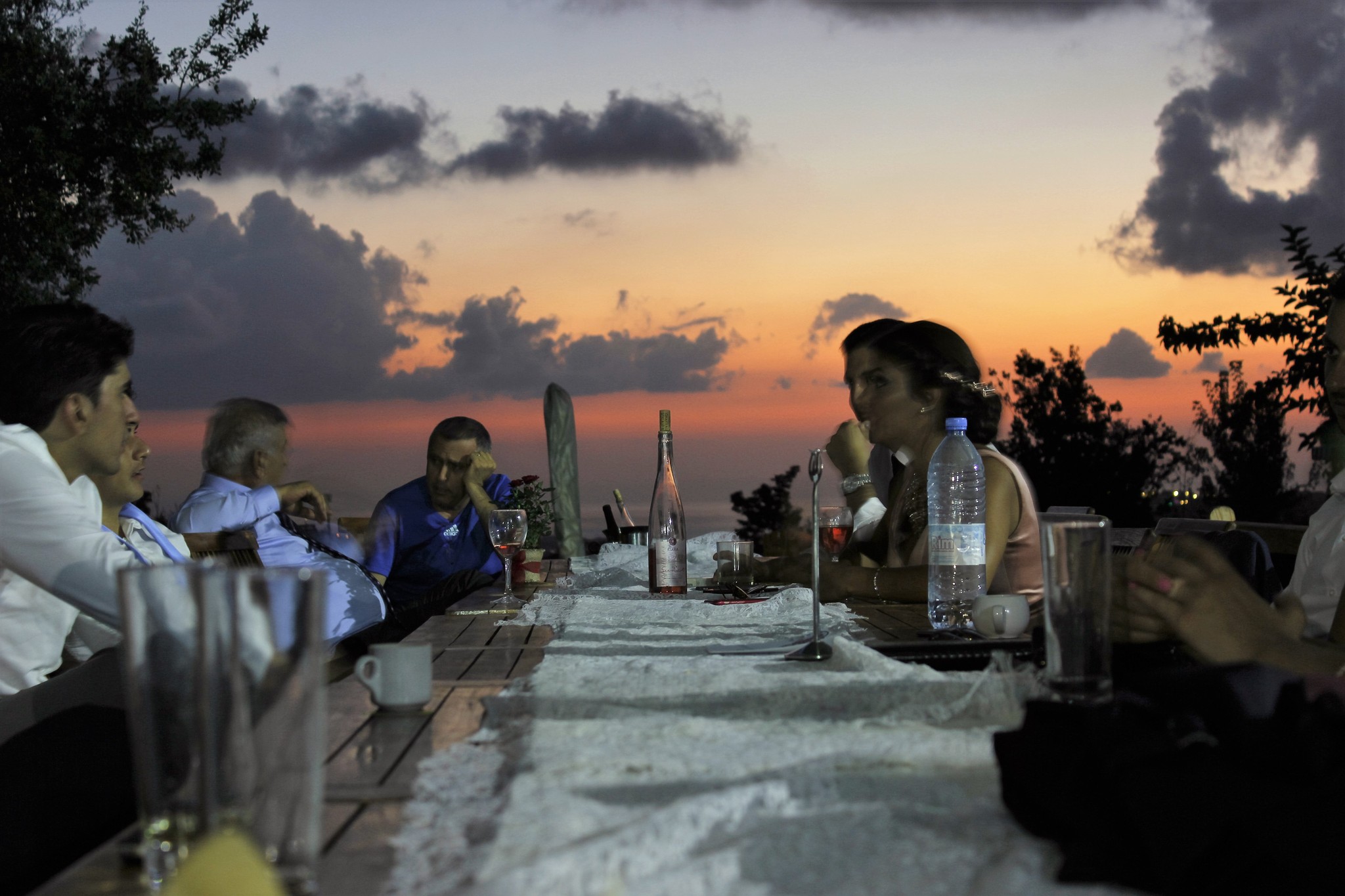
[904,468,927,531]
[117,513,173,567]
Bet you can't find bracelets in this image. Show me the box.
[874,565,888,603]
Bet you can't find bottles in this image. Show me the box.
[926,417,987,630]
[603,504,626,543]
[613,489,637,526]
[648,409,687,594]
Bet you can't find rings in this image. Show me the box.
[477,454,480,456]
[1158,577,1182,599]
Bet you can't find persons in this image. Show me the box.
[294,490,331,523]
[710,318,1045,672]
[1092,264,1345,677]
[168,398,407,660]
[0,299,285,896]
[366,417,518,632]
[64,380,275,679]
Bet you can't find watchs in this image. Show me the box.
[840,474,872,495]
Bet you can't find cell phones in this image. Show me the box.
[704,597,771,606]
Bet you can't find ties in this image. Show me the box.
[885,454,907,508]
[274,507,401,625]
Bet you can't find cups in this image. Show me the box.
[119,563,330,896]
[717,541,755,586]
[973,593,1030,639]
[357,641,433,710]
[1038,512,1112,698]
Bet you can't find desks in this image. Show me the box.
[38,556,1145,896]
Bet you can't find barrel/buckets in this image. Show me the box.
[602,525,648,547]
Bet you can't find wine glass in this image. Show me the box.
[488,510,528,604]
[819,506,853,563]
